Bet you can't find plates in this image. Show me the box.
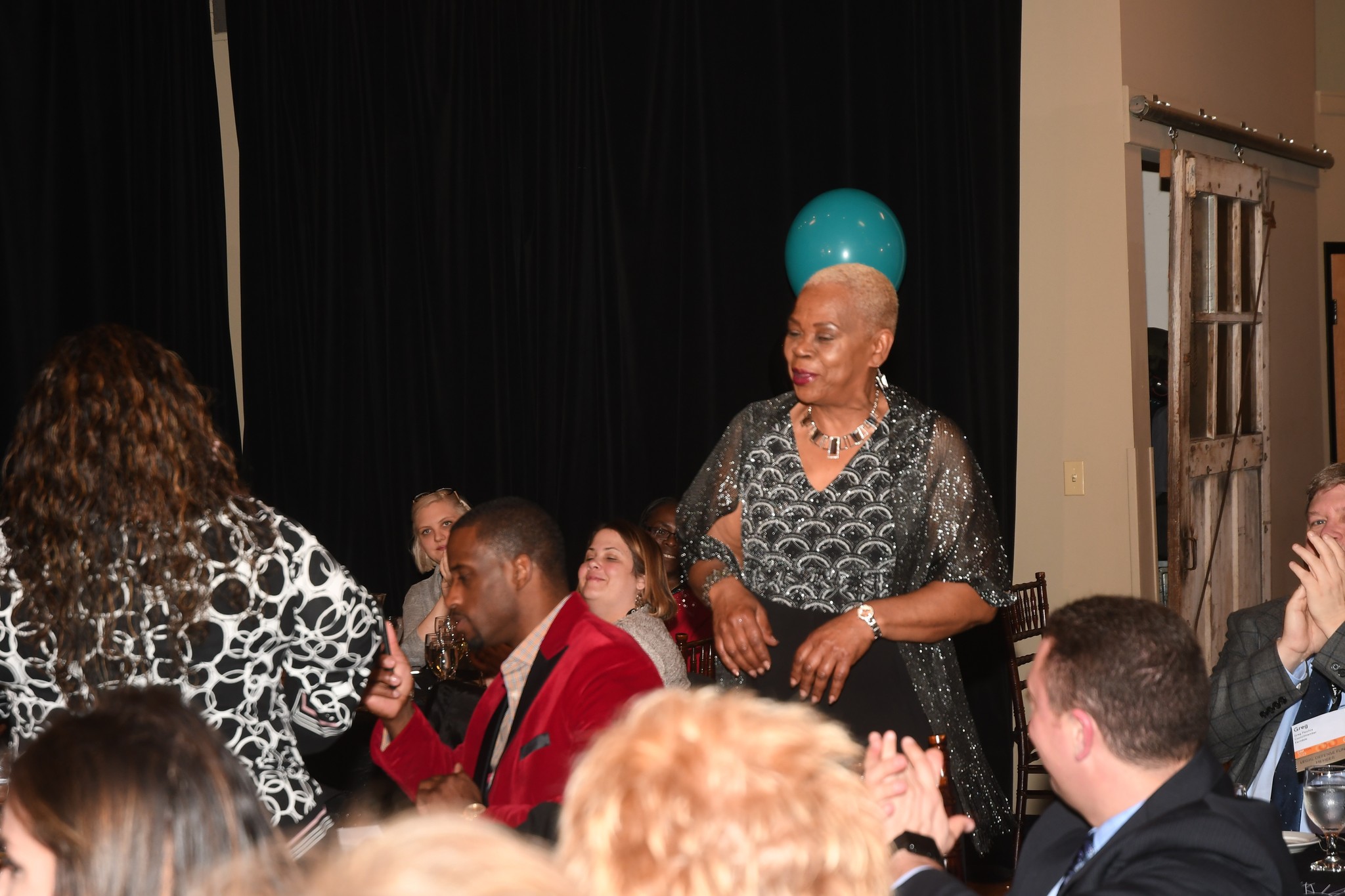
[1282,831,1321,853]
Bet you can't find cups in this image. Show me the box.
[1234,783,1247,798]
[424,615,468,681]
[386,616,404,646]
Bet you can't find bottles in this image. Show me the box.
[370,593,389,654]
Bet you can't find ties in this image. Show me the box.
[1270,668,1336,832]
[1057,834,1094,892]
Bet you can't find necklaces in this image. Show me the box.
[800,385,880,459]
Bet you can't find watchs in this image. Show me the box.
[463,803,486,821]
[889,831,946,867]
[857,604,882,640]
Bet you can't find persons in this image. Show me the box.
[674,263,1015,875]
[640,496,714,674]
[555,686,896,896]
[0,685,300,896]
[863,594,1298,896]
[577,520,692,690]
[1206,462,1345,834]
[399,488,472,668]
[0,321,384,880]
[362,497,663,829]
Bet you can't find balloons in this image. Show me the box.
[785,188,907,298]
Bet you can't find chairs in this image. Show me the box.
[1003,571,1053,875]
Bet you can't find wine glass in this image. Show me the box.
[478,670,486,688]
[1303,765,1345,872]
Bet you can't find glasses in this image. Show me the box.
[412,488,461,503]
[645,525,679,542]
